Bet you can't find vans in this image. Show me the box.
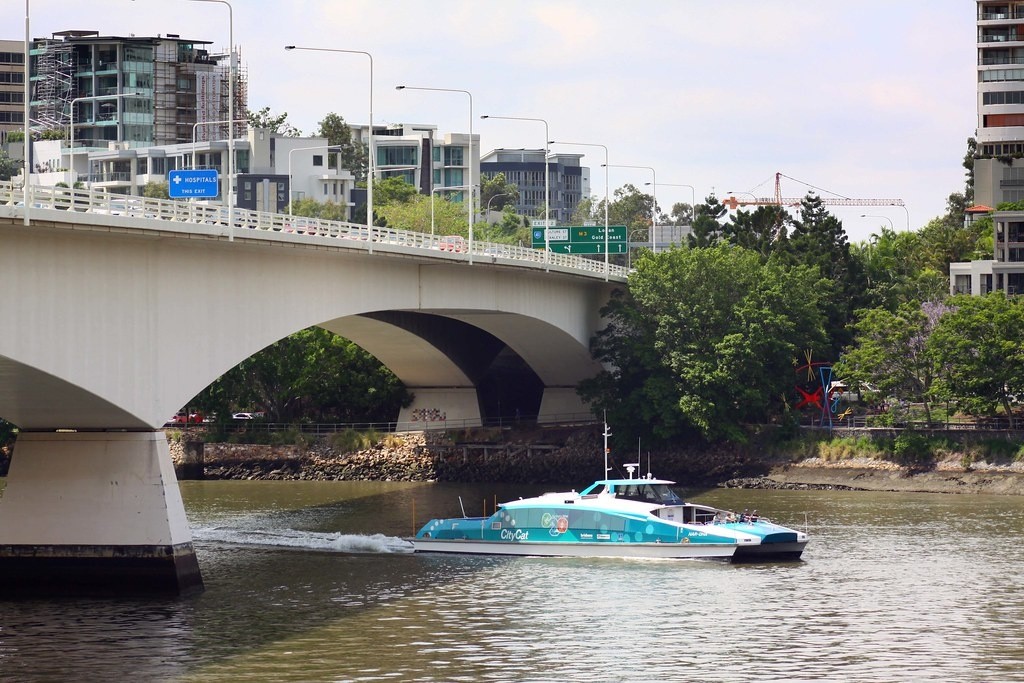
[826,380,883,402]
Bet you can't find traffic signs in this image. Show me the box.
[531,226,628,254]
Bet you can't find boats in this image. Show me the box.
[401,408,810,562]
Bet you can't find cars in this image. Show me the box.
[282,219,325,237]
[382,234,418,248]
[205,208,255,229]
[85,199,155,219]
[484,246,509,259]
[16,201,56,210]
[343,230,375,242]
[438,236,468,253]
[167,411,263,423]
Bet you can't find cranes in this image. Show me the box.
[722,172,906,241]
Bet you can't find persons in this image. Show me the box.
[713,511,723,525]
[739,509,759,522]
[725,511,739,523]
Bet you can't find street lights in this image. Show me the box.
[601,164,655,255]
[285,46,373,255]
[628,229,649,271]
[548,141,609,283]
[367,167,418,241]
[645,183,695,222]
[396,86,473,255]
[288,144,342,216]
[431,184,479,247]
[69,92,140,189]
[727,192,758,211]
[487,193,511,243]
[191,119,255,203]
[481,116,550,273]
